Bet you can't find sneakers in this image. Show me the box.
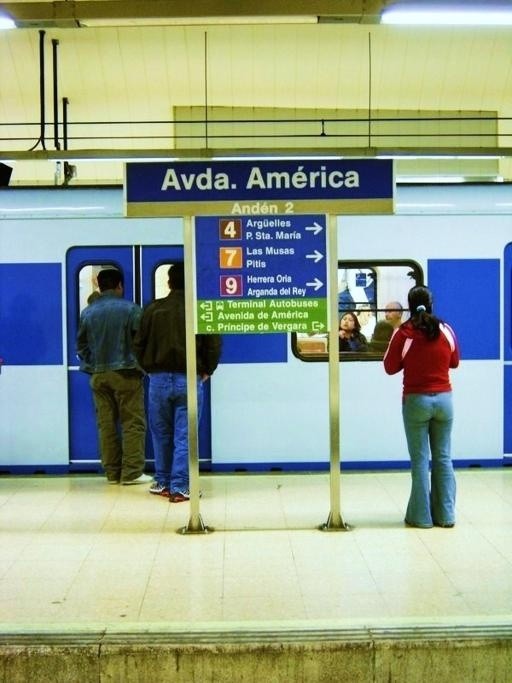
[149,484,190,503]
[108,475,155,484]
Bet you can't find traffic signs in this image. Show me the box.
[194,214,329,333]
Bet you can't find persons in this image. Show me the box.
[133,263,222,502]
[383,286,460,529]
[76,268,154,485]
[369,321,394,352]
[326,313,368,352]
[385,302,403,328]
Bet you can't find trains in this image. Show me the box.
[0,176,512,477]
